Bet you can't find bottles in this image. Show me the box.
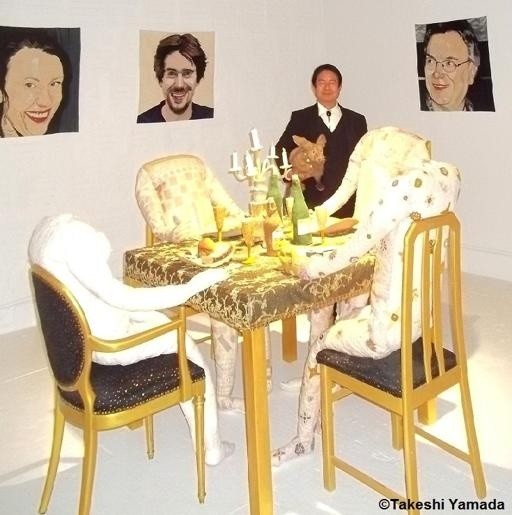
[263,197,286,256]
[266,174,283,221]
[291,174,312,246]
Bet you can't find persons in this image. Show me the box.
[421,19,481,111]
[0,27,73,137]
[272,64,367,219]
[137,34,213,123]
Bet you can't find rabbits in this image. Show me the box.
[287,134,328,191]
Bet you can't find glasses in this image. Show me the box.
[423,51,472,72]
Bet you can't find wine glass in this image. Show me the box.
[213,203,264,264]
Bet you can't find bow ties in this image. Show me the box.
[326,112,331,117]
